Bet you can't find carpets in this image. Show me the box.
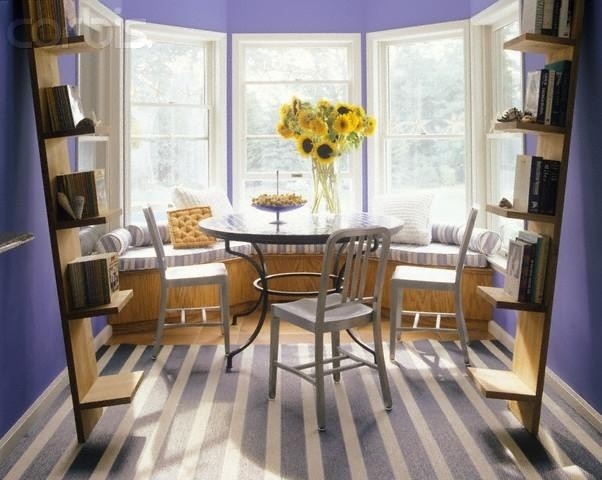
[0,341,601,480]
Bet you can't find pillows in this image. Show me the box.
[386,206,435,245]
[97,227,133,257]
[166,204,218,248]
[125,222,171,247]
[430,218,500,255]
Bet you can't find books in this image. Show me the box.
[502,229,550,304]
[67,252,121,310]
[34,0,75,41]
[59,167,110,218]
[510,154,559,215]
[45,83,86,131]
[520,0,573,40]
[523,60,570,126]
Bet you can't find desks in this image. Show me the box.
[196,201,399,376]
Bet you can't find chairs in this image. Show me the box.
[390,204,484,367]
[136,200,234,369]
[267,226,397,436]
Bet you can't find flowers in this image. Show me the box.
[276,92,375,166]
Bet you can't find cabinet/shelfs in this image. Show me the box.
[12,1,148,449]
[467,0,591,442]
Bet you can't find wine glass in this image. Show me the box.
[251,204,304,224]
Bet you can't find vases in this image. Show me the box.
[311,155,340,217]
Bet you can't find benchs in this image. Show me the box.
[81,215,495,332]
